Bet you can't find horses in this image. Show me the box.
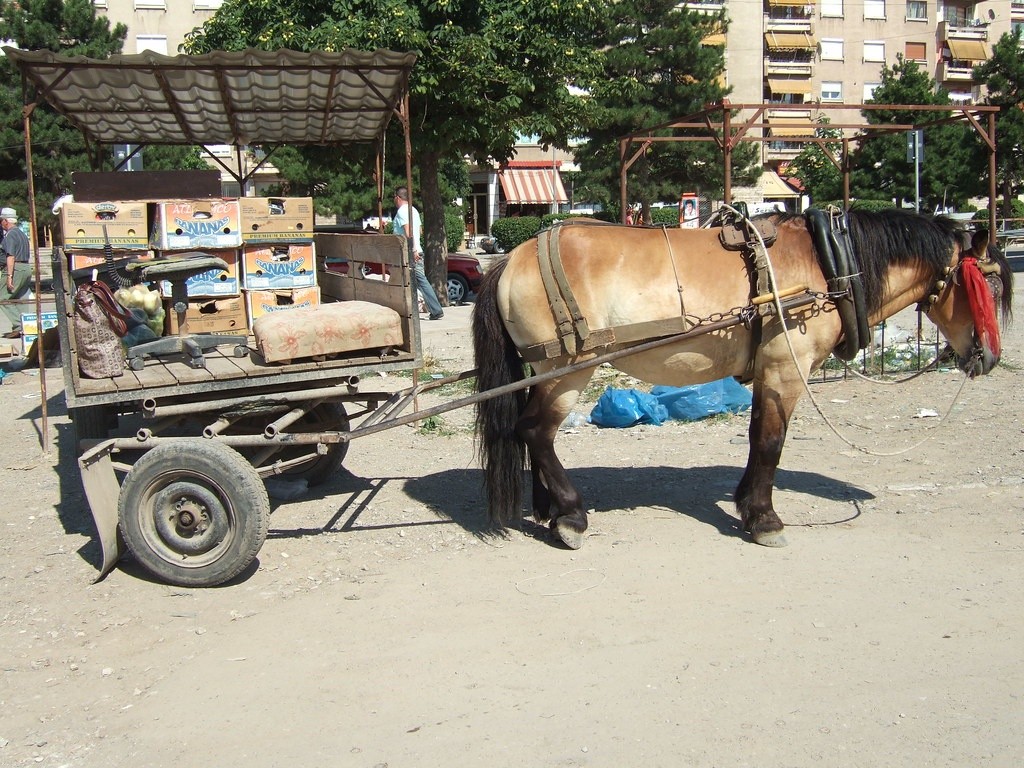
[471,206,1015,550]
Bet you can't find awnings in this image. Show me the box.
[497,159,573,206]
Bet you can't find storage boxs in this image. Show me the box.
[68,168,224,205]
[148,198,244,250]
[56,200,151,253]
[240,242,319,291]
[68,250,157,290]
[236,195,315,245]
[20,334,39,357]
[19,311,58,335]
[164,297,251,341]
[244,285,323,335]
[157,246,243,299]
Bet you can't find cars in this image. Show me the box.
[476,234,508,257]
[307,225,490,309]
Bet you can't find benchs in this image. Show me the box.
[252,299,404,365]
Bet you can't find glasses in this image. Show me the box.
[392,195,397,199]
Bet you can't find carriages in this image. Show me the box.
[1,22,1014,587]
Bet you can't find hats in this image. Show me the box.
[0,208,18,218]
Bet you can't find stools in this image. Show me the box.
[125,250,249,370]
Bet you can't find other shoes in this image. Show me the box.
[429,309,444,321]
[2,331,21,338]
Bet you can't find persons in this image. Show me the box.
[392,187,443,321]
[635,212,644,226]
[683,200,698,228]
[626,208,635,226]
[0,206,32,338]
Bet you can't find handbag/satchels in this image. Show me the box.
[71,279,132,378]
